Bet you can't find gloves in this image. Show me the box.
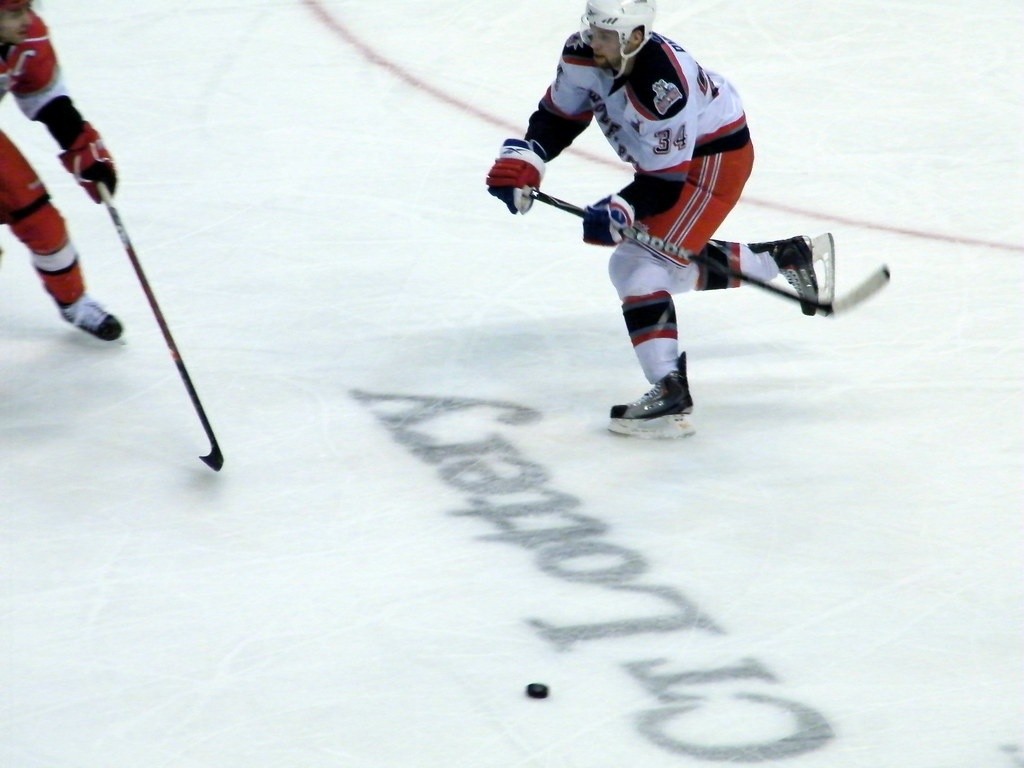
[581,193,635,247]
[486,138,548,214]
[57,120,117,203]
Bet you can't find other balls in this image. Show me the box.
[527,683,549,699]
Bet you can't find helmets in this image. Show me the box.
[586,0,656,44]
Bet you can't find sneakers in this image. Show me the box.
[747,233,834,318]
[61,294,123,345]
[607,352,694,439]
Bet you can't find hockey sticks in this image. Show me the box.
[530,189,893,319]
[97,180,226,476]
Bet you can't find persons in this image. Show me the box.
[0,0,123,342]
[485,0,821,424]
